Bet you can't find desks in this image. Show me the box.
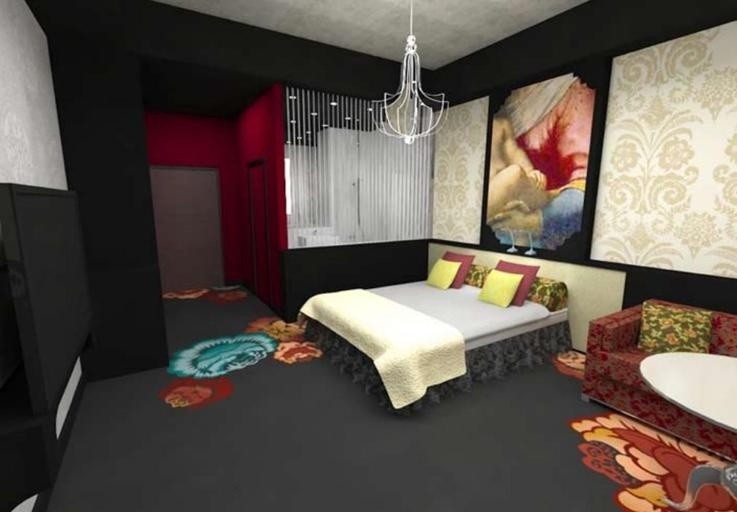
[637,347,736,436]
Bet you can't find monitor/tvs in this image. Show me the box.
[0,183,91,418]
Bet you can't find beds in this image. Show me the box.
[295,262,570,424]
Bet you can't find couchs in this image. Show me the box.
[579,293,735,472]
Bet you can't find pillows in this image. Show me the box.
[633,297,713,355]
[427,249,570,316]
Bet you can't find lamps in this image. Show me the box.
[370,0,452,150]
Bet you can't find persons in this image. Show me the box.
[487,117,550,225]
